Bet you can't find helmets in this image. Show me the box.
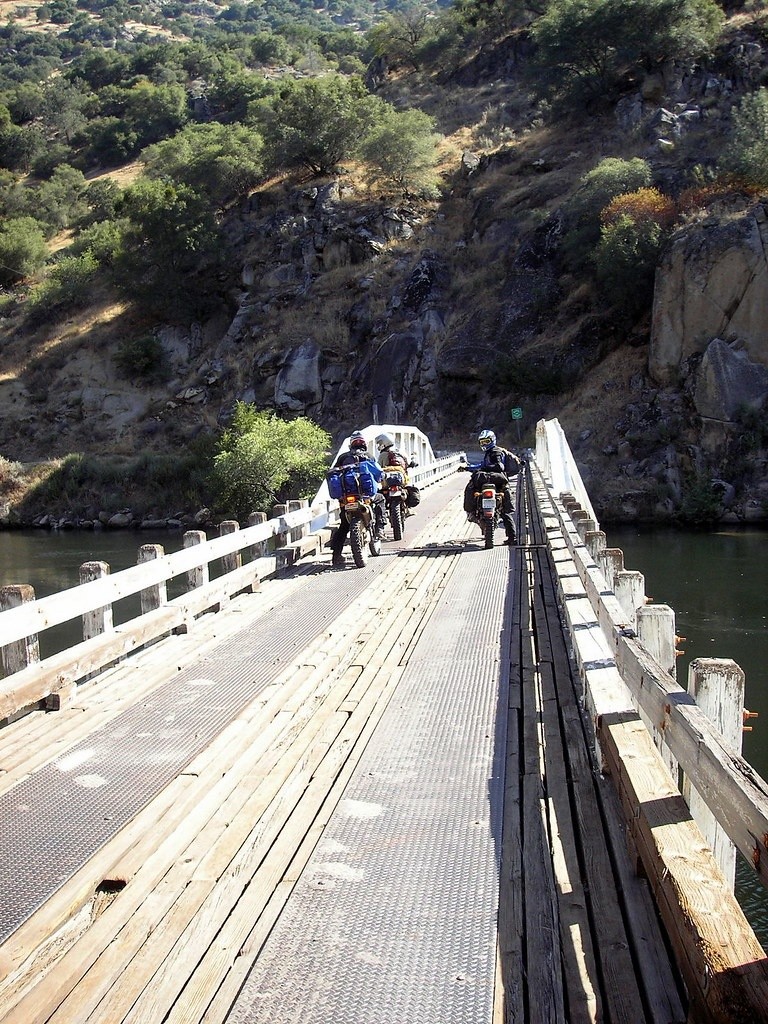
[375,433,394,453]
[478,430,496,451]
[351,438,367,452]
[349,431,364,440]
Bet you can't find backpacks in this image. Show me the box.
[384,448,406,471]
[497,446,522,477]
[342,452,365,466]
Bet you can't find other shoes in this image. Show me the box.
[332,555,347,565]
[467,512,476,522]
[376,528,389,539]
[504,537,517,546]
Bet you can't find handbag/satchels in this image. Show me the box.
[377,488,390,510]
[405,486,421,507]
[326,461,376,499]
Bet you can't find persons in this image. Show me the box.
[330,431,387,562]
[372,432,417,522]
[465,430,517,545]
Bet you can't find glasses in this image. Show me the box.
[480,439,491,445]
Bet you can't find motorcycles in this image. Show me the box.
[339,473,387,568]
[458,455,526,550]
[376,461,418,541]
